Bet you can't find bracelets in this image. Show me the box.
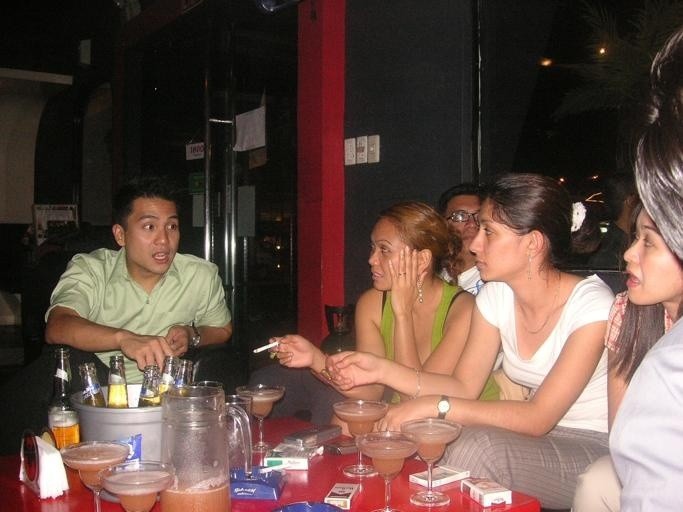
[436,394,450,420]
[308,367,330,387]
[408,366,421,400]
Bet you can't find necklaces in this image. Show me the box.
[516,272,561,334]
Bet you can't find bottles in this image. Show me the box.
[48,348,80,451]
[77,354,193,407]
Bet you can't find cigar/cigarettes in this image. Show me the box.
[252,340,280,354]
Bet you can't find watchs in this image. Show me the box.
[190,322,201,350]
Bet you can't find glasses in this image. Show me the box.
[446,210,480,226]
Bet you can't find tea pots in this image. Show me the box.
[158,388,253,511]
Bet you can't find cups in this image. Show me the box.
[97,459,175,511]
[195,380,252,466]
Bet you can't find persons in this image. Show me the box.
[433,183,502,372]
[325,173,616,509]
[586,172,642,295]
[39,176,248,404]
[605,35,682,511]
[267,202,498,459]
[570,195,683,512]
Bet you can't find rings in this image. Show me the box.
[398,272,406,275]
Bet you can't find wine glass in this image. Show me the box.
[59,440,129,511]
[330,398,388,478]
[400,417,462,507]
[235,385,285,452]
[354,432,421,512]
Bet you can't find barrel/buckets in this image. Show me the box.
[66,383,163,504]
